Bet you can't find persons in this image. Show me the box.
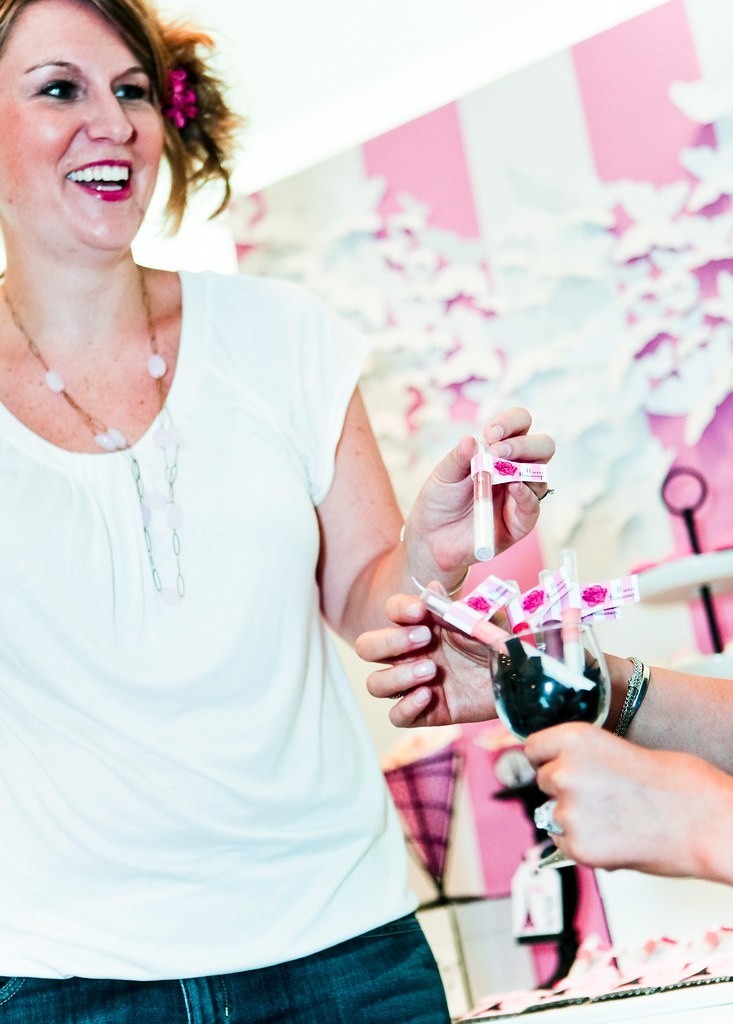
[0,2,554,1024]
[358,580,733,884]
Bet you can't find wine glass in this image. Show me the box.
[488,624,612,871]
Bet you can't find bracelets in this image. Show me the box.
[614,657,642,737]
[628,662,650,721]
[399,525,470,595]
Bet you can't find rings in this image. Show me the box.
[531,802,565,835]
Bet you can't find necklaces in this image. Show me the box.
[1,265,185,600]
